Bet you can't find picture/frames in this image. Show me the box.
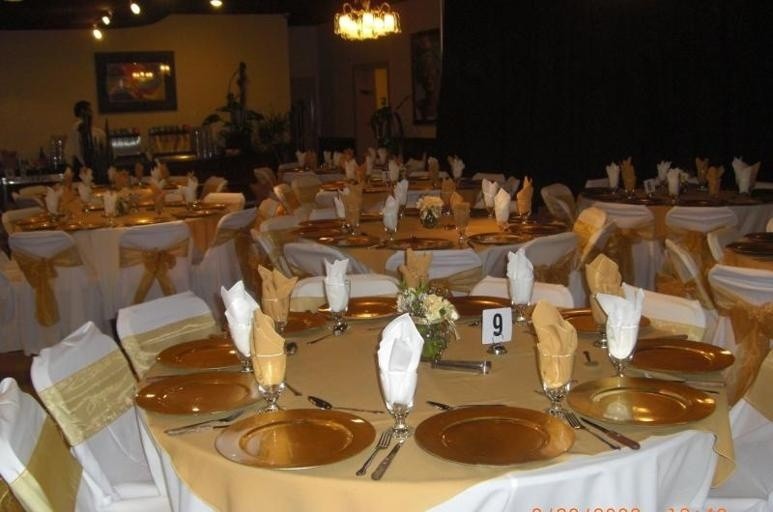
[94,50,178,113]
[410,28,442,126]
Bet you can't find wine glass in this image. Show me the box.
[591,291,622,350]
[318,277,358,336]
[232,326,263,377]
[504,272,534,329]
[603,322,634,378]
[254,354,285,411]
[533,345,571,419]
[376,370,421,438]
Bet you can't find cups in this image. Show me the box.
[260,295,293,336]
[3,154,33,182]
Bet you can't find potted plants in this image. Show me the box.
[202,93,264,151]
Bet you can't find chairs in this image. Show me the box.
[0,162,773,512]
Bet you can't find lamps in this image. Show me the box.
[333,0,401,42]
[101,10,114,25]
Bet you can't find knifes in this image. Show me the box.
[577,414,639,452]
[374,431,411,484]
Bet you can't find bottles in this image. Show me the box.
[37,144,50,172]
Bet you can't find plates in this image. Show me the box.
[719,229,772,257]
[215,407,378,471]
[159,340,250,371]
[11,190,229,230]
[411,403,574,463]
[557,298,650,336]
[128,372,267,419]
[293,203,571,252]
[568,373,716,426]
[626,339,735,377]
[288,163,479,193]
[574,179,757,206]
[439,292,513,316]
[323,295,402,323]
[286,311,329,334]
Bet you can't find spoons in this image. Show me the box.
[306,391,386,418]
[578,343,602,369]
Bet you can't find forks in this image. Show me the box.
[560,410,622,454]
[355,425,394,474]
